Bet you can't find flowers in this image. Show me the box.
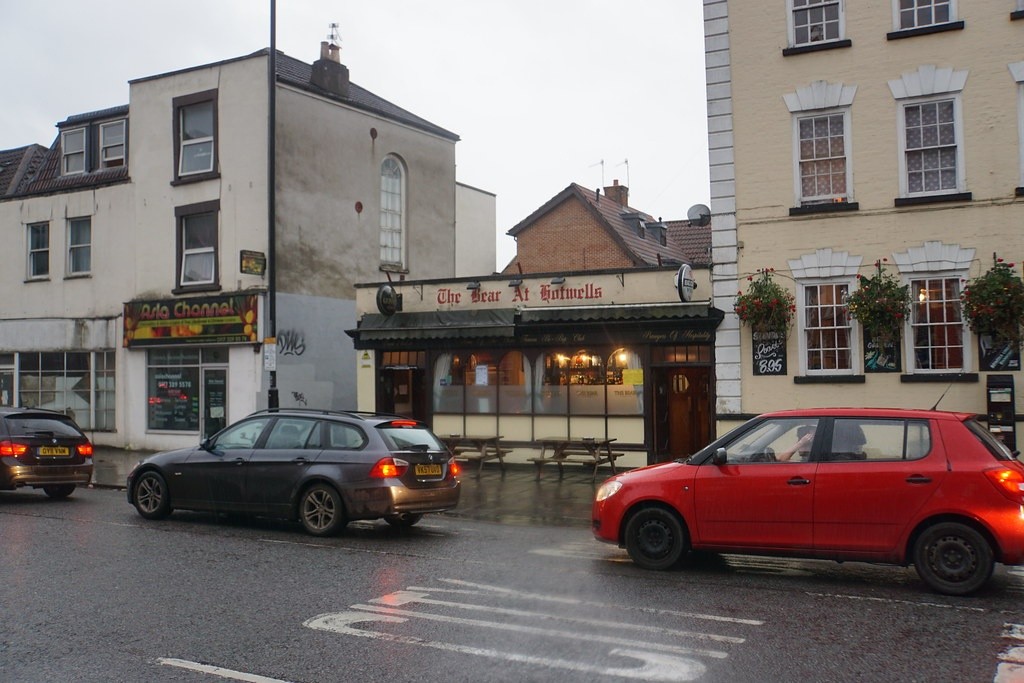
[843,257,914,359]
[959,257,1024,354]
[734,267,796,348]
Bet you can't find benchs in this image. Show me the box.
[527,458,601,485]
[559,451,625,475]
[450,448,513,482]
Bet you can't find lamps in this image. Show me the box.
[508,280,523,287]
[466,280,481,290]
[550,276,565,284]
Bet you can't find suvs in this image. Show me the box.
[125,407,462,536]
[0,405,93,497]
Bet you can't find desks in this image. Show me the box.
[438,434,507,481]
[535,436,618,483]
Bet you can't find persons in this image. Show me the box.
[777,426,816,463]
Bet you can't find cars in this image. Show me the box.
[591,408,1024,595]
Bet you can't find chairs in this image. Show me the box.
[273,425,302,450]
[833,423,868,460]
